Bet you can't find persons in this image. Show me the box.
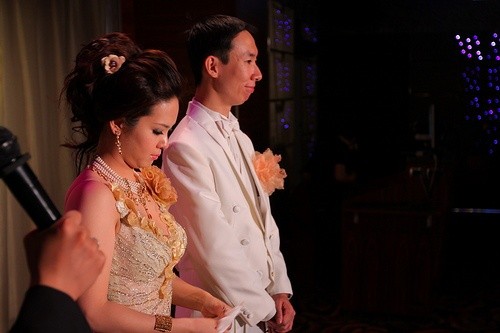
[8,210,105,333]
[56,34,236,333]
[160,17,296,333]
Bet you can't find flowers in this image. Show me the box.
[141,166,178,207]
[250,148,287,195]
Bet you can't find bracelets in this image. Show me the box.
[152,313,174,333]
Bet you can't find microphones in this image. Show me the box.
[0,126,62,230]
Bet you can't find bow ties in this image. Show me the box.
[216,114,240,139]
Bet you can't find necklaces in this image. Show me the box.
[89,153,155,220]
